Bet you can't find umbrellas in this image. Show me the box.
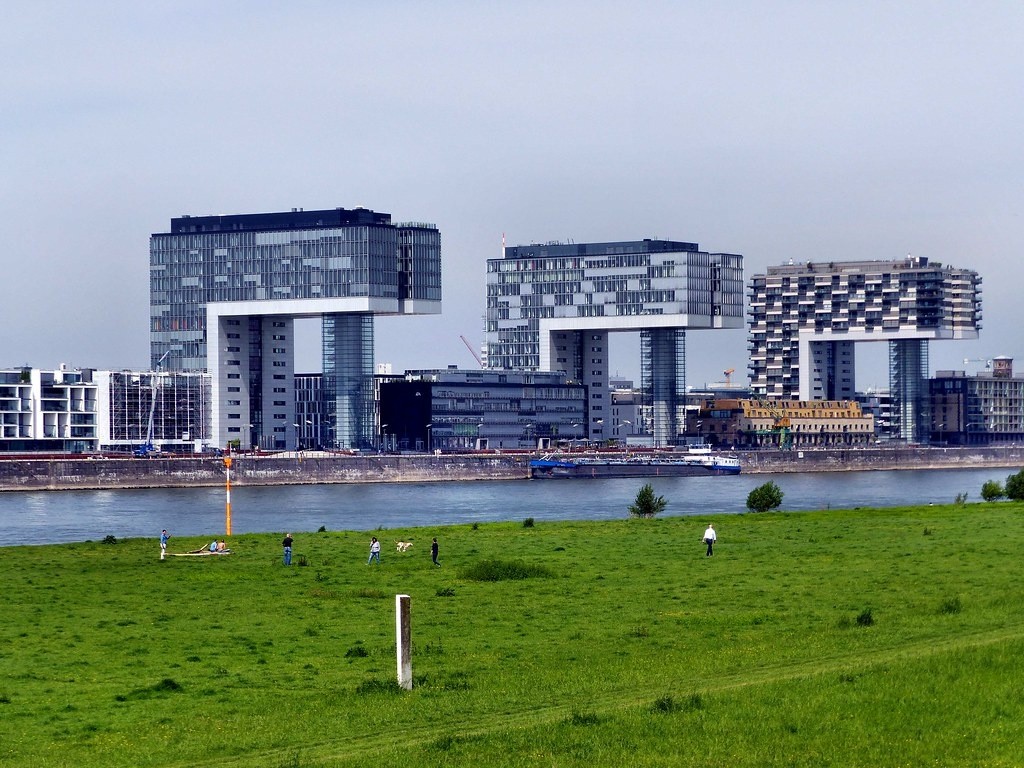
[558,437,605,448]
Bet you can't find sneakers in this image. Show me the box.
[364,563,372,566]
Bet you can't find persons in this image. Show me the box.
[161,530,172,559]
[210,538,230,552]
[282,534,293,565]
[365,537,381,565]
[211,449,220,459]
[703,525,716,555]
[431,538,441,568]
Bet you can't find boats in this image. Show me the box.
[528,439,742,481]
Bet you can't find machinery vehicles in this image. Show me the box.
[132,350,177,459]
[736,383,793,450]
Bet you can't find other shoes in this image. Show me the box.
[437,565,441,567]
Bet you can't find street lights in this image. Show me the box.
[241,424,255,458]
[939,423,944,448]
[476,424,483,438]
[306,419,312,451]
[426,424,432,454]
[382,424,388,455]
[525,424,532,450]
[597,419,605,453]
[572,423,580,453]
[292,423,301,452]
[695,420,704,450]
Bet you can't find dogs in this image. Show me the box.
[394,539,414,553]
